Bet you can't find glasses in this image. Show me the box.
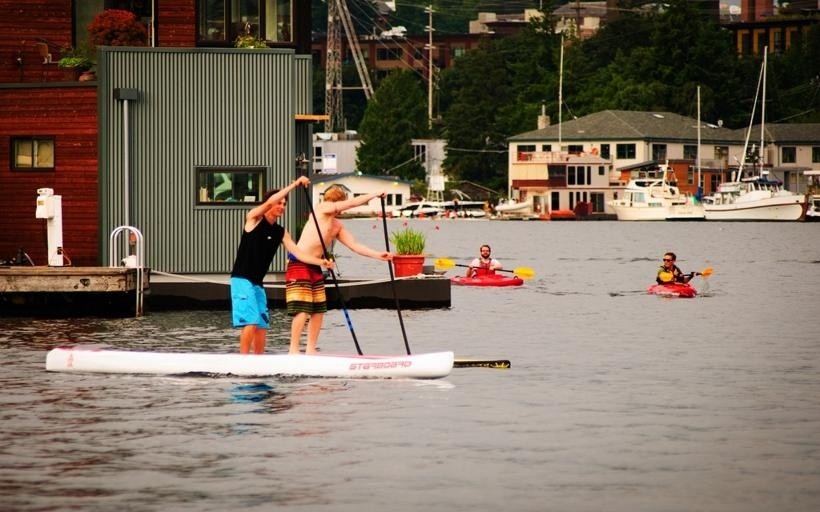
[662,258,673,263]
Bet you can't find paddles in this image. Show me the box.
[659,268,714,282]
[433,257,537,279]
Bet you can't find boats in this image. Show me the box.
[647,280,698,299]
[452,274,525,287]
[604,158,704,223]
[806,195,820,223]
[42,348,457,381]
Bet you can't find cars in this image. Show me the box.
[398,202,441,217]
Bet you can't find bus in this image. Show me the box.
[438,199,488,218]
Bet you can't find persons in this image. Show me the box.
[656,253,697,284]
[466,245,503,277]
[286,184,393,354]
[231,176,334,354]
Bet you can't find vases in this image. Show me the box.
[79,71,96,81]
[123,255,136,267]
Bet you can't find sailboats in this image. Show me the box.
[704,43,807,223]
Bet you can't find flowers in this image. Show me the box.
[89,10,147,45]
[130,234,137,255]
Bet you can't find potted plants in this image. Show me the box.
[389,228,425,277]
[58,57,91,81]
